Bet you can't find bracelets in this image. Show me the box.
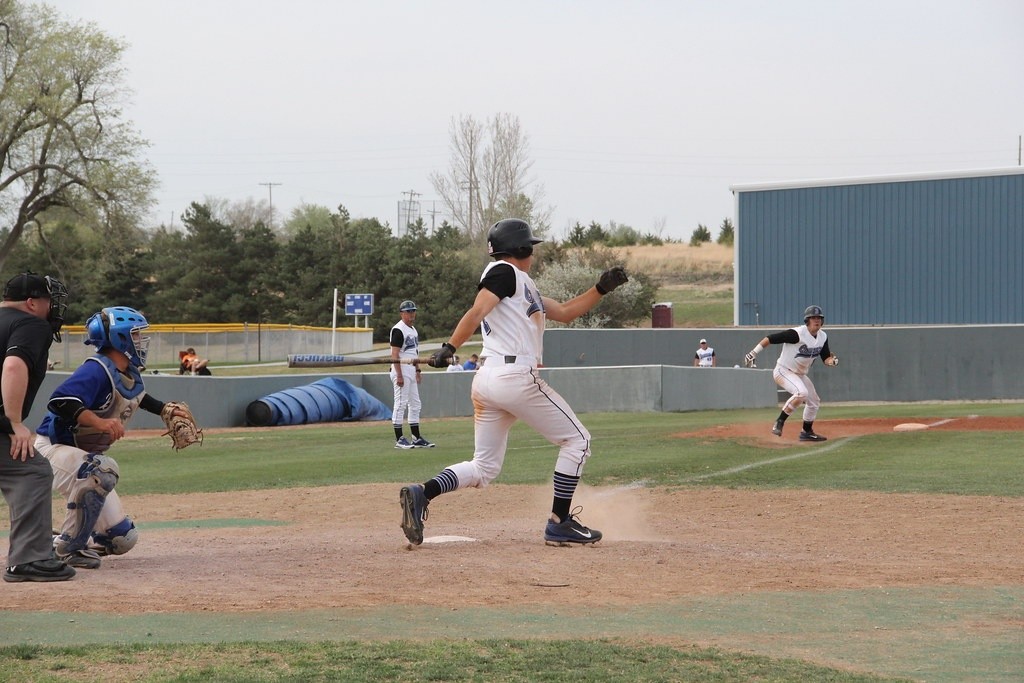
[416,369,421,372]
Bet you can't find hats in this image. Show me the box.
[700,338,706,344]
[399,300,418,311]
[4,273,49,301]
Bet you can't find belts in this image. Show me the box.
[505,356,517,362]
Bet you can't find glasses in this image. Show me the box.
[702,342,706,344]
[406,309,416,313]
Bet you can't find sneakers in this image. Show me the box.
[394,436,415,449]
[412,437,435,448]
[800,431,827,441]
[3,558,75,582]
[53,547,100,569]
[399,484,429,545]
[545,506,602,546]
[52,530,62,548]
[772,419,784,436]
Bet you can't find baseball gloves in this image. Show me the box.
[159,400,205,454]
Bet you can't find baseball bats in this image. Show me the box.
[286,352,461,368]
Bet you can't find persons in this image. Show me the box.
[400,218,629,547]
[463,354,480,370]
[694,339,716,367]
[35,306,200,569]
[745,305,839,442]
[0,270,76,582]
[389,301,436,449]
[183,348,208,376]
[447,356,464,371]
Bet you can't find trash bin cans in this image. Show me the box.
[652,302,673,328]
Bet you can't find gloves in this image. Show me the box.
[829,352,839,366]
[427,342,456,368]
[745,352,757,368]
[595,267,628,295]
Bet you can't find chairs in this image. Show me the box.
[179,351,189,375]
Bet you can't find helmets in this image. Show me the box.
[486,218,544,258]
[85,305,151,370]
[803,305,824,325]
